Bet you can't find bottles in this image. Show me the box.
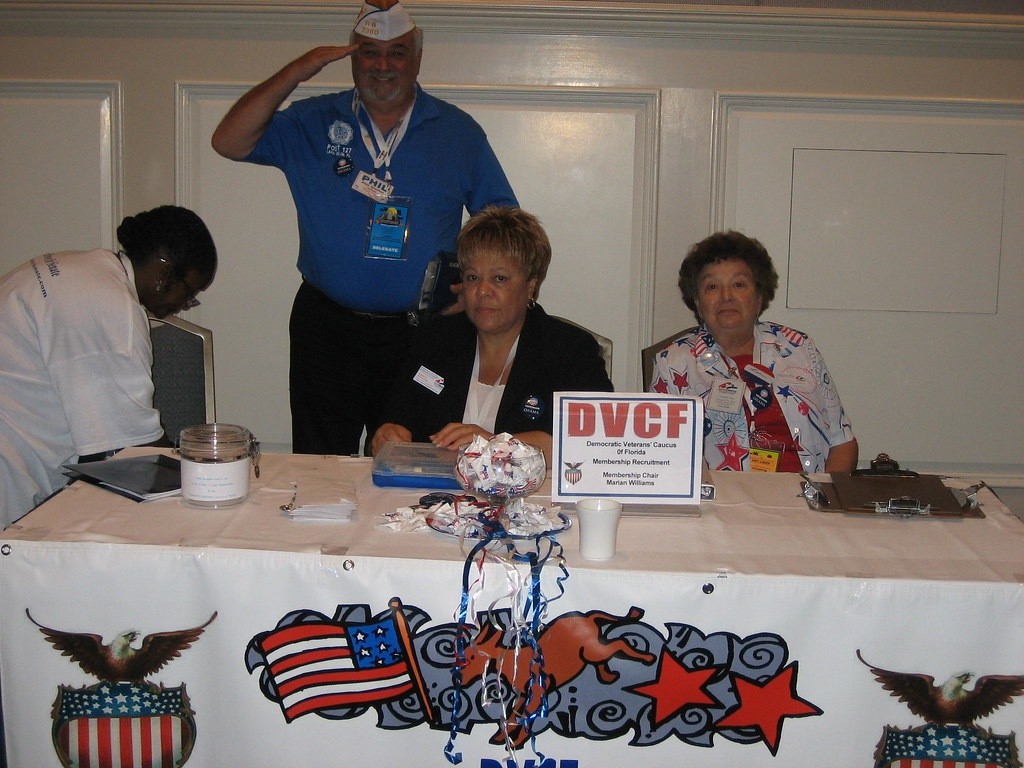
[173,424,260,506]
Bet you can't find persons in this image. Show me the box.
[649,230,859,472]
[210,0,521,456]
[363,207,614,469]
[0,203,217,531]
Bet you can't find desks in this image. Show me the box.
[0,442,1024,768]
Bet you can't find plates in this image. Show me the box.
[426,505,572,541]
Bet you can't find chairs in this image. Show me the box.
[145,308,217,444]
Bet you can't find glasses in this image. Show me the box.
[160,257,201,308]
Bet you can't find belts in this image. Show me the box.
[308,281,407,325]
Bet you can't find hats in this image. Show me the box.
[351,0,417,41]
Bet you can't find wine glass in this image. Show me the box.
[454,444,546,536]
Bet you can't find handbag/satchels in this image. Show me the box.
[418,251,461,313]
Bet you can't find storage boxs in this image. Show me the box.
[371,442,465,491]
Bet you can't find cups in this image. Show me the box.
[576,499,622,561]
